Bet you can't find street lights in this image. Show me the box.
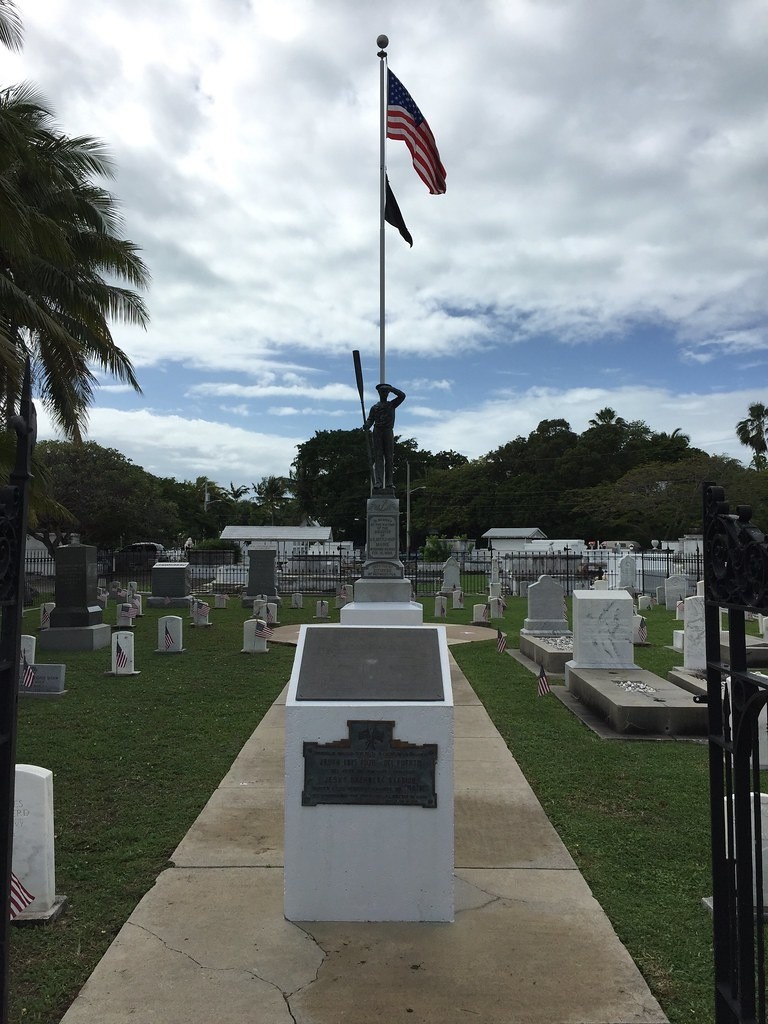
[406,486,428,561]
[204,500,222,512]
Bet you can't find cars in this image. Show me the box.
[326,561,341,576]
[399,556,424,562]
[577,562,607,579]
[112,542,167,574]
[599,541,641,549]
[350,559,365,569]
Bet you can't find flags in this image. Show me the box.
[386,70,447,195]
[164,597,171,605]
[638,618,647,642]
[23,659,34,687]
[538,667,550,696]
[341,587,348,599]
[164,628,173,648]
[384,178,413,246]
[197,603,211,617]
[322,603,328,616]
[498,600,504,613]
[267,608,273,624]
[42,609,50,625]
[458,594,462,603]
[122,607,137,619]
[97,582,136,609]
[132,596,139,607]
[441,606,445,616]
[498,631,506,652]
[116,644,127,668]
[255,622,273,639]
[483,607,488,619]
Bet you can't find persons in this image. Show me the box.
[364,385,406,489]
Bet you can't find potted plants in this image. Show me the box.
[186,538,243,566]
